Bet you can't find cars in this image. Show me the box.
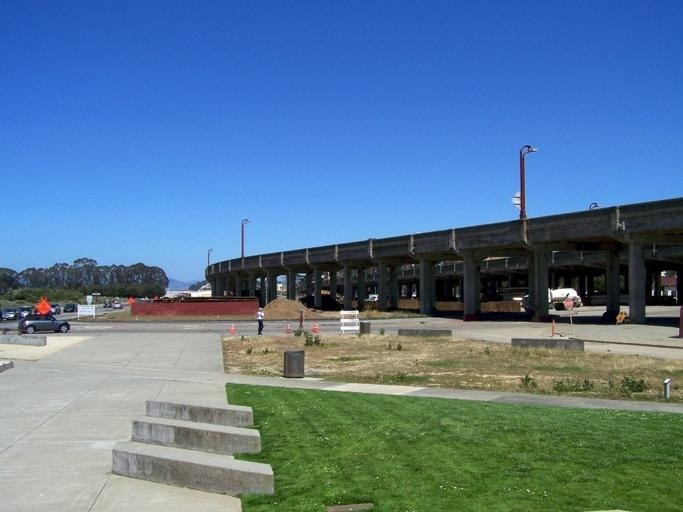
[16,305,33,320]
[103,300,112,307]
[114,301,122,308]
[18,312,71,334]
[1,307,16,320]
[175,292,191,300]
[50,303,61,315]
[64,303,77,312]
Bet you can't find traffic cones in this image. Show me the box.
[312,321,320,333]
[287,323,292,333]
[229,323,235,334]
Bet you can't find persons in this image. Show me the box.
[104,298,108,308]
[298,310,305,329]
[256,308,264,335]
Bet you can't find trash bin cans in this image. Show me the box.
[360,321,370,336]
[284,350,305,378]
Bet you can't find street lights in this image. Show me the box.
[241,218,250,267]
[518,143,538,243]
[207,248,214,268]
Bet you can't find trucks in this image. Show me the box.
[523,288,582,310]
